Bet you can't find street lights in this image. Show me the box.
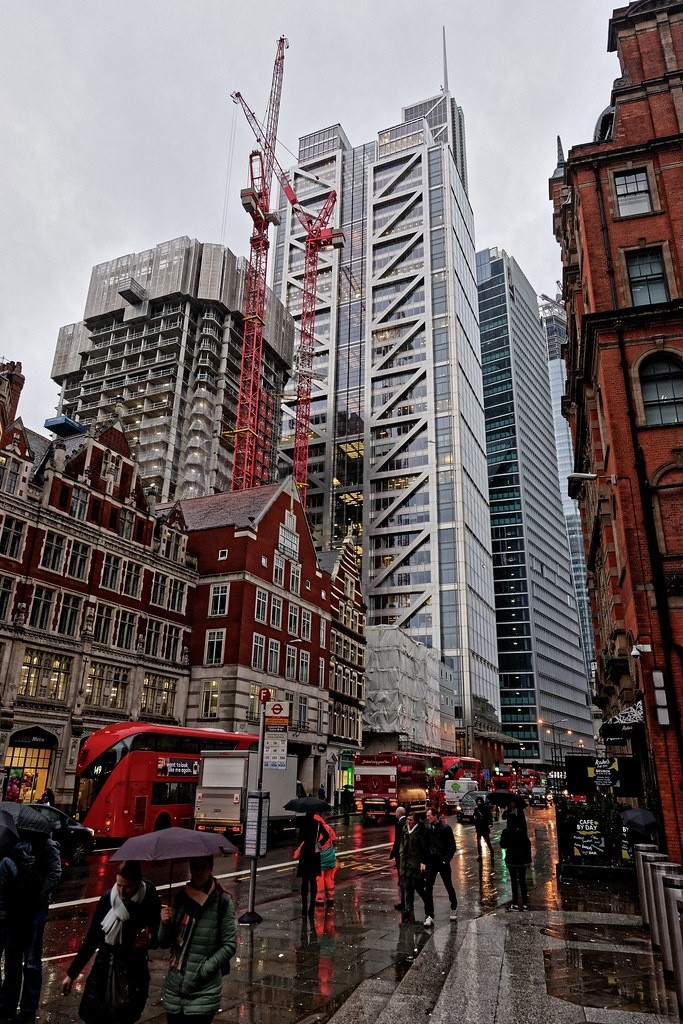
[552,718,582,777]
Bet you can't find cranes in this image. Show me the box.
[227,32,350,512]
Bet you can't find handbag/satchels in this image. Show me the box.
[320,845,336,870]
[315,843,322,853]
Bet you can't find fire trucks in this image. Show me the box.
[353,750,444,824]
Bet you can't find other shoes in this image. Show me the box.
[315,901,324,906]
[424,914,434,926]
[450,909,457,920]
[506,904,520,912]
[308,910,314,920]
[302,908,306,918]
[394,903,405,910]
[523,905,529,912]
[328,900,334,905]
[401,914,415,922]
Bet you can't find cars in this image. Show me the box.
[456,791,501,825]
[20,804,97,867]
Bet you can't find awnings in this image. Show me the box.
[599,700,645,739]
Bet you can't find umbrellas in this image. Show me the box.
[283,797,332,813]
[486,790,528,810]
[620,808,657,834]
[108,827,238,906]
[0,801,58,859]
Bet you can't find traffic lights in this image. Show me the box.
[512,761,518,772]
[0,705,15,731]
[494,759,500,772]
[71,714,84,737]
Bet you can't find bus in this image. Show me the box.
[71,722,259,850]
[442,756,539,814]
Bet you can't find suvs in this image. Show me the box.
[529,785,554,809]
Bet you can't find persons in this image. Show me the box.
[0,828,62,1024]
[500,819,532,912]
[292,812,338,918]
[42,787,54,806]
[294,917,335,1016]
[472,797,495,860]
[62,860,160,1024]
[502,801,527,831]
[390,806,458,926]
[158,855,237,1024]
[318,783,326,800]
